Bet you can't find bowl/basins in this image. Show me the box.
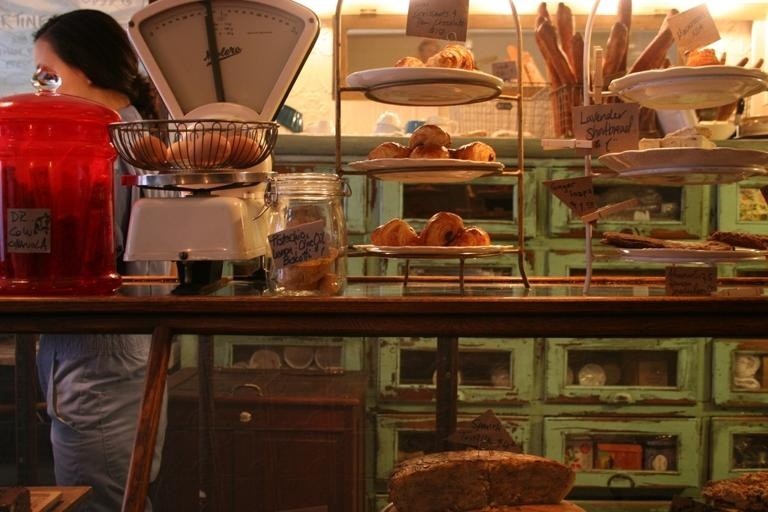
[700,119,736,140]
[373,111,402,135]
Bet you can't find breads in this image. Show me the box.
[367,124,496,162]
[388,44,477,71]
[388,449,576,512]
[507,0,768,251]
[371,211,492,245]
[703,471,768,512]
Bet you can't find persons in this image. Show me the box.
[30,9,170,512]
[415,38,440,63]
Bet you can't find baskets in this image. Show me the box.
[106,118,280,173]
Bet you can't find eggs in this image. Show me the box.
[131,132,262,167]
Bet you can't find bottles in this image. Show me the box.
[252,169,353,299]
[1,63,127,298]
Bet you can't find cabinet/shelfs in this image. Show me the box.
[0,0,768,512]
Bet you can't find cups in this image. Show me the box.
[406,114,459,136]
[735,353,762,377]
[738,115,768,136]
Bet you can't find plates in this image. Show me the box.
[355,240,508,262]
[596,146,768,186]
[607,63,768,111]
[248,345,342,371]
[565,361,624,385]
[612,243,764,264]
[344,157,505,186]
[345,66,505,108]
